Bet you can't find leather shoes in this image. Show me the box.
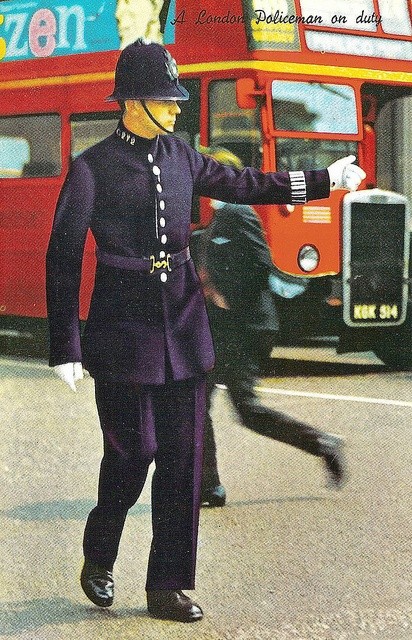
[147,588,202,622]
[80,554,113,605]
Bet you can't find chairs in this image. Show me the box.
[20,163,55,178]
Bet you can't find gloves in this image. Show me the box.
[46,362,84,387]
[327,155,366,191]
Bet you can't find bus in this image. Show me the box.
[1,0,412,372]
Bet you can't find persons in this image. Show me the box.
[45,36,367,623]
[201,147,348,507]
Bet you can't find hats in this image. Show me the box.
[103,42,190,104]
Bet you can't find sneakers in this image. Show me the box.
[198,485,224,508]
[324,436,348,489]
